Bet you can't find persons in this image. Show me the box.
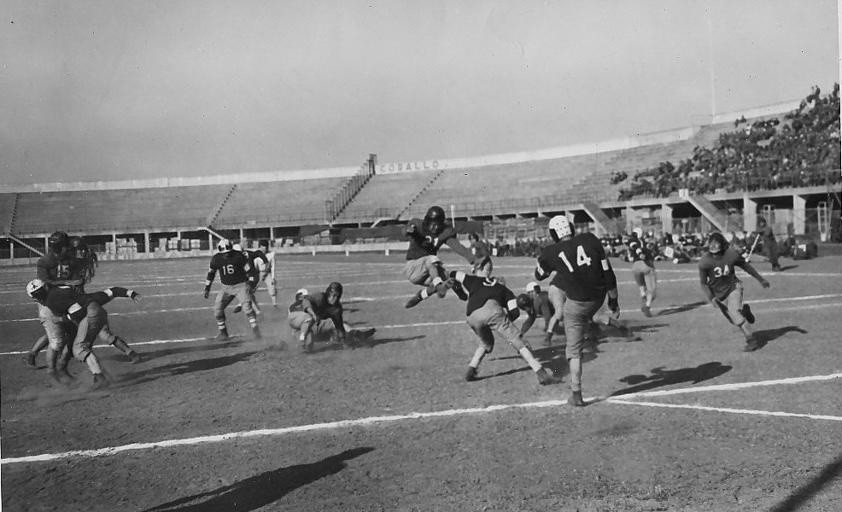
[405,207,492,309]
[535,215,620,407]
[628,227,657,318]
[783,233,798,258]
[609,81,841,204]
[699,233,771,351]
[599,230,763,264]
[26,231,144,393]
[495,235,556,258]
[518,282,641,347]
[752,216,781,271]
[807,237,818,257]
[288,282,377,354]
[449,270,563,384]
[202,239,278,342]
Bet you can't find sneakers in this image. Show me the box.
[43,369,109,394]
[543,332,553,346]
[567,391,584,406]
[27,353,36,368]
[741,304,754,323]
[536,368,556,384]
[582,297,652,352]
[253,327,261,338]
[120,352,141,365]
[465,365,477,381]
[404,278,468,309]
[212,332,230,341]
[233,303,243,313]
[742,332,767,352]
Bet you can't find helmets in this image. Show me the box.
[424,206,493,277]
[296,287,309,297]
[632,227,644,239]
[708,232,727,255]
[526,282,540,295]
[217,238,233,252]
[233,243,243,251]
[518,294,532,312]
[24,230,93,299]
[549,214,576,240]
[326,281,344,306]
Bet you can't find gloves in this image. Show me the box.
[608,296,621,320]
[712,297,723,309]
[761,280,770,290]
[128,289,144,303]
[204,289,208,299]
